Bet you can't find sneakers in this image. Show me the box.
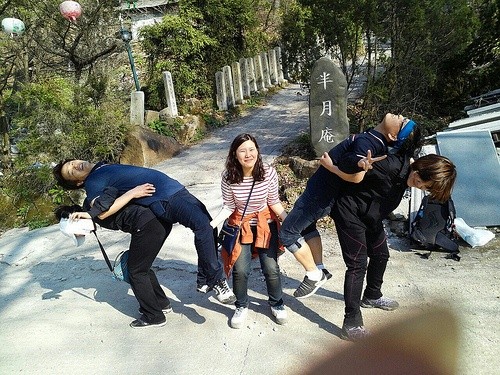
[293,269,327,298]
[322,268,333,280]
[213,281,237,305]
[196,284,213,294]
[130,316,167,329]
[339,325,366,341]
[231,307,248,329]
[271,304,287,318]
[138,303,173,315]
[361,296,399,311]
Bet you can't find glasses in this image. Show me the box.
[397,116,409,139]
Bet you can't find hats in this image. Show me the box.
[58,218,101,247]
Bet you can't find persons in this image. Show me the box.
[53,159,237,304]
[330,150,457,343]
[279,113,427,299]
[210,133,288,329]
[55,197,172,328]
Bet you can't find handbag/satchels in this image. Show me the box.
[217,218,240,256]
[112,250,131,284]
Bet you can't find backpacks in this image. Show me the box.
[410,195,459,259]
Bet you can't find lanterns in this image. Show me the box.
[59,1,81,22]
[2,18,25,37]
